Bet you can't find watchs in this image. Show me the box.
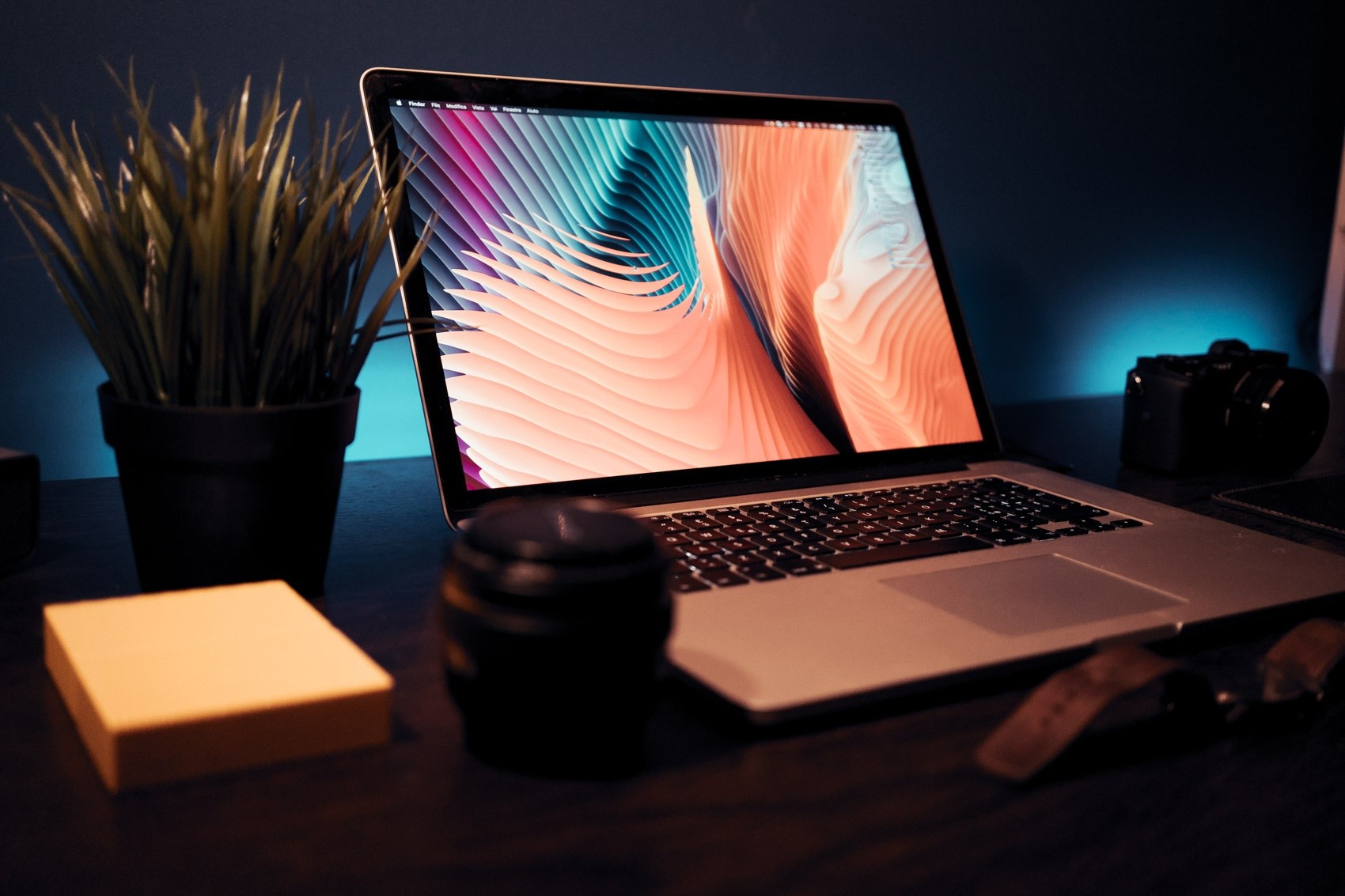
[974,618,1345,786]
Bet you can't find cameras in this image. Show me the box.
[1125,337,1328,474]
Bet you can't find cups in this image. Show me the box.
[434,504,675,785]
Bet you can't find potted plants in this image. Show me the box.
[0,61,438,593]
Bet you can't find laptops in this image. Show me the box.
[356,67,1345,727]
